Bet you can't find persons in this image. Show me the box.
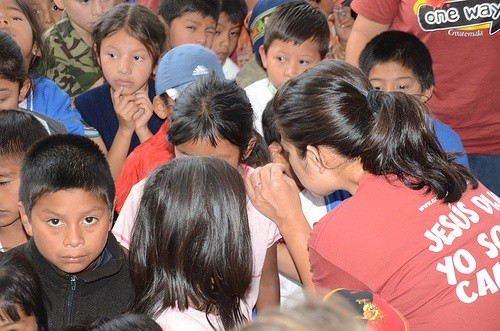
[0,251,48,331]
[115,44,225,214]
[0,133,148,331]
[243,2,332,137]
[113,75,281,321]
[1,0,355,152]
[345,0,500,197]
[129,156,254,331]
[359,30,469,168]
[75,3,167,182]
[244,59,500,331]
[0,109,50,252]
[262,99,354,284]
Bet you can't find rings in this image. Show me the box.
[253,181,263,190]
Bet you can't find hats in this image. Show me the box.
[155,44,226,100]
